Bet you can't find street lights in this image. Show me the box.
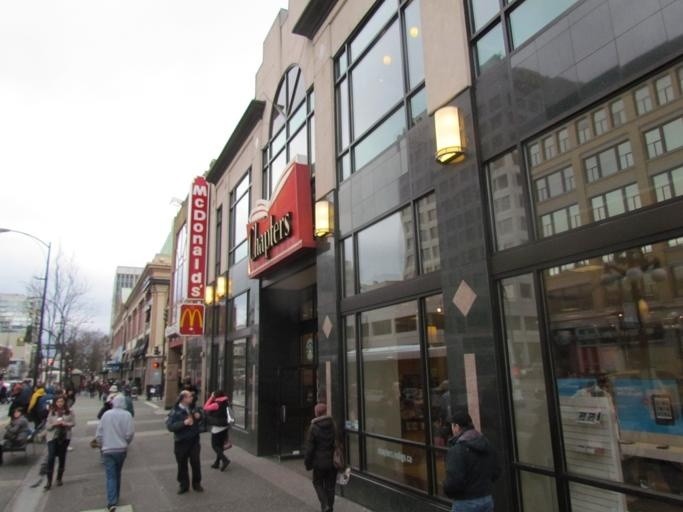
[26,295,63,388]
[601,247,668,434]
[0,226,50,389]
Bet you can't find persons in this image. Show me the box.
[441,411,495,511]
[304,402,346,511]
[0,374,237,511]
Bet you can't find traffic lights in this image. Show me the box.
[151,362,158,369]
[152,346,159,356]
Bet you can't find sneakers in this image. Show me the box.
[334,440,347,474]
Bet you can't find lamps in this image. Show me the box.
[215,274,227,300]
[311,197,332,240]
[431,104,468,171]
[202,285,214,305]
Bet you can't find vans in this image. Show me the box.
[602,369,682,422]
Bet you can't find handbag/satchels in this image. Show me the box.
[226,407,235,424]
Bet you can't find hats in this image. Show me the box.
[315,404,327,414]
[446,411,472,424]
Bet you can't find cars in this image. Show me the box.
[534,379,593,400]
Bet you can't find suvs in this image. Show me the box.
[520,363,532,378]
[427,384,465,409]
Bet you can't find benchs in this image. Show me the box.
[0,420,48,465]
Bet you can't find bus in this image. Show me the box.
[459,329,524,410]
